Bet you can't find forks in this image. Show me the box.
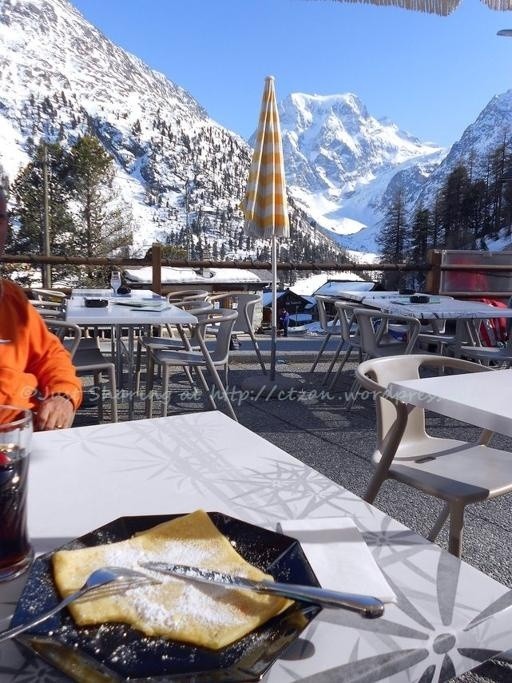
[1,564,149,643]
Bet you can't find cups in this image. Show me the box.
[0,403,35,583]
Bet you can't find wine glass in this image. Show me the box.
[111,270,121,296]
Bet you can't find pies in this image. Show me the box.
[51,512,293,652]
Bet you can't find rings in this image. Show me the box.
[54,422,65,429]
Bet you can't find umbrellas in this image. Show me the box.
[243,74,288,381]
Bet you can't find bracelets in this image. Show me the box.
[46,390,75,412]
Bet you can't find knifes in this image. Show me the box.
[137,559,384,618]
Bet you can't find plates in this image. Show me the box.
[6,509,326,680]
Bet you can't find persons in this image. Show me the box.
[1,190,85,433]
